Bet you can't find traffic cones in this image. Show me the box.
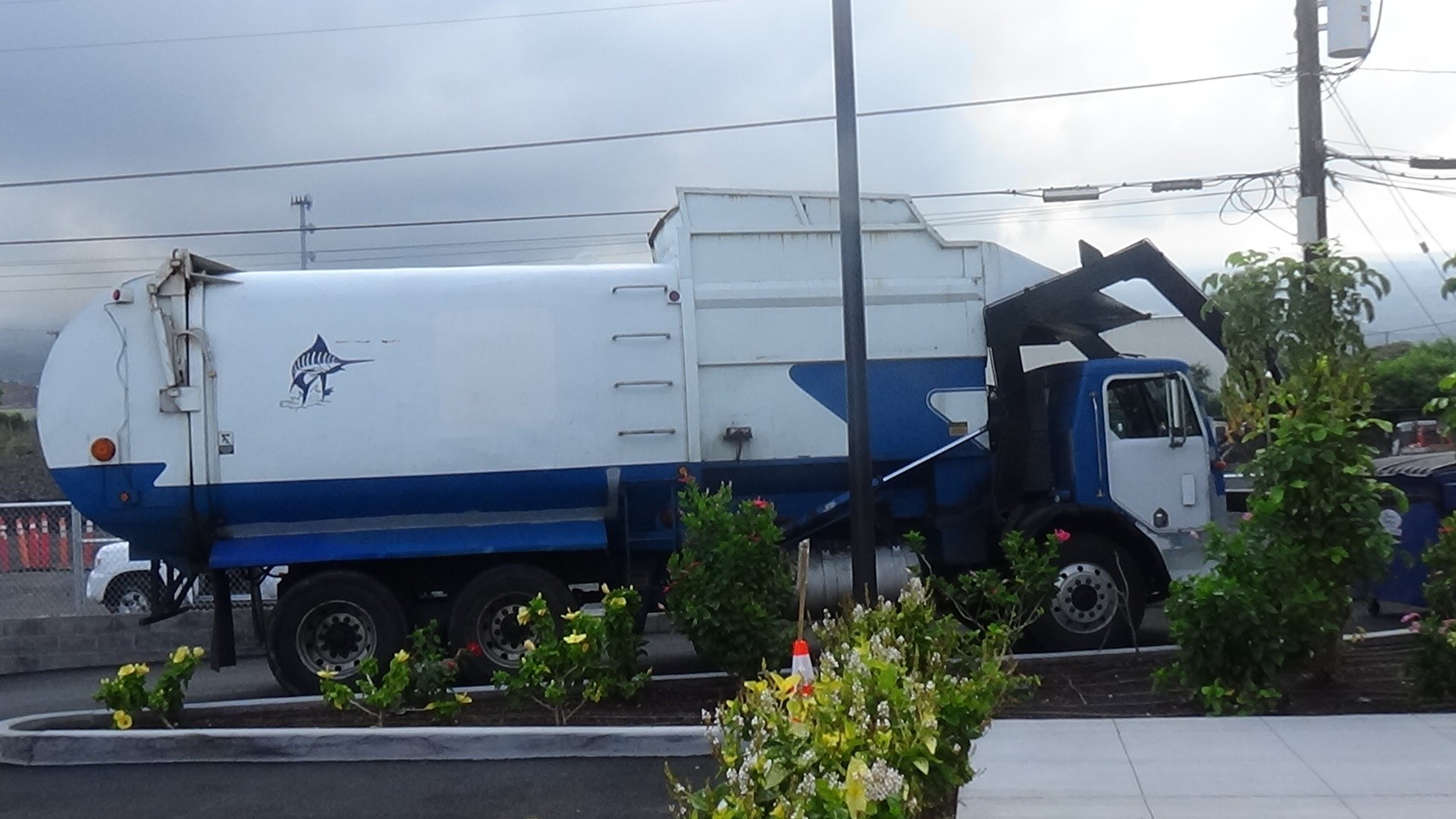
[785,639,818,727]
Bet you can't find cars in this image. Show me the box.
[84,539,290,616]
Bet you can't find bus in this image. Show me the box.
[1390,420,1456,456]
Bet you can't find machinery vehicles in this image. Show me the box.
[33,185,1287,697]
